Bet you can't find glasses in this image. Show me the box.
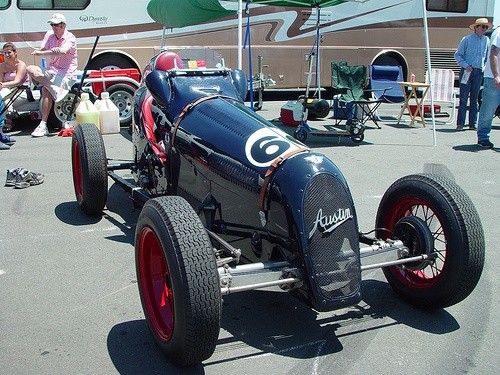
[49,23,62,27]
[478,25,488,29]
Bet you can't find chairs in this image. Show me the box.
[420,68,456,125]
[154,51,185,71]
[329,61,413,129]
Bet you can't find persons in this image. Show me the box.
[26,13,78,137]
[0,42,31,150]
[454,18,491,131]
[477,26,500,149]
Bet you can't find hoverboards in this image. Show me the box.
[294,52,365,142]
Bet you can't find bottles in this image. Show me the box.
[94,92,120,134]
[75,93,100,133]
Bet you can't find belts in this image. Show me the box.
[473,68,482,71]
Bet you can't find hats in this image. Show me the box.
[47,14,67,24]
[468,18,494,30]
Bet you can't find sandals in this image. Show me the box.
[478,139,493,147]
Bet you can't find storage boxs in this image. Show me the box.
[409,104,441,116]
[281,101,308,127]
[90,68,140,95]
[333,93,356,119]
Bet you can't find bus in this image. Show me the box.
[0,0,494,93]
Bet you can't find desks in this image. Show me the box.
[395,82,430,129]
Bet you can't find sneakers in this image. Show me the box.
[15,169,45,189]
[6,168,24,186]
[31,124,49,137]
[50,85,69,103]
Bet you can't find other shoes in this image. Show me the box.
[469,125,477,131]
[456,125,463,130]
[0,134,16,143]
[0,142,11,150]
